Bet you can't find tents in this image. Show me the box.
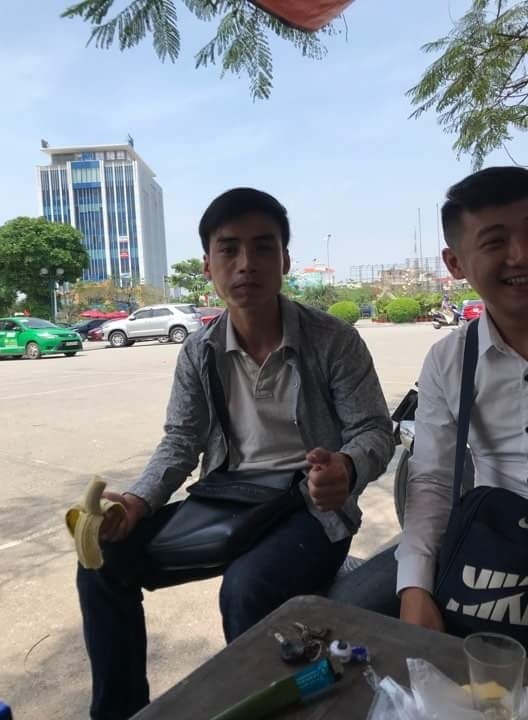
[79,308,130,319]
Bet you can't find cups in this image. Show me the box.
[462,631,526,720]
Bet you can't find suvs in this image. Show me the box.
[358,303,372,318]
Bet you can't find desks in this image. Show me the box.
[129,596,528,720]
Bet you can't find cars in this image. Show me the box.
[0,317,85,360]
[100,303,226,348]
[459,299,486,322]
[55,319,108,342]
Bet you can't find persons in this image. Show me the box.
[76,188,392,720]
[388,167,528,635]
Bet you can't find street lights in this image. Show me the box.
[327,234,332,288]
[40,267,64,322]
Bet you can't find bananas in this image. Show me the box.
[64,475,128,569]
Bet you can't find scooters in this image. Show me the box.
[430,303,461,330]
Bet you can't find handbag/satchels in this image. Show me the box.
[149,466,304,571]
[432,486,528,649]
